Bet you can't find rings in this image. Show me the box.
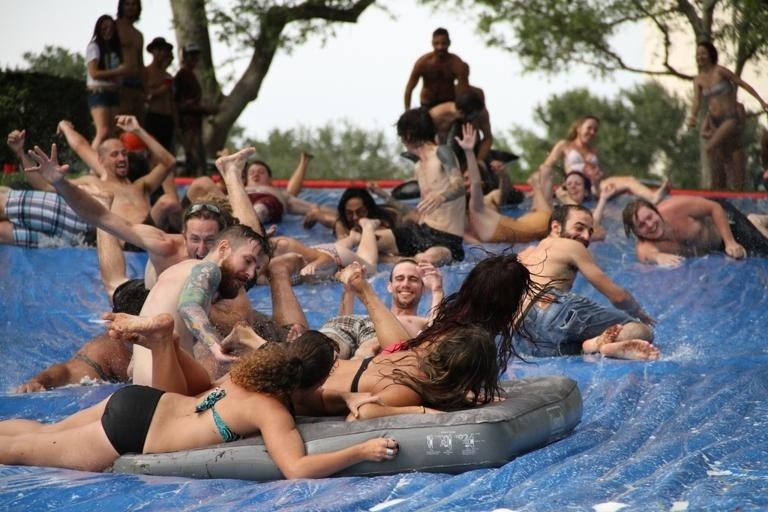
[386,441,388,448]
[387,449,394,456]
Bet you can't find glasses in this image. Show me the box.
[182,202,220,214]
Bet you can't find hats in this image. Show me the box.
[182,42,202,54]
[146,37,173,53]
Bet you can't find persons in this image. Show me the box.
[453,62,485,143]
[426,90,495,173]
[86,0,220,178]
[403,28,468,109]
[689,43,767,191]
[0,329,398,479]
[1,107,768,428]
[701,79,750,188]
